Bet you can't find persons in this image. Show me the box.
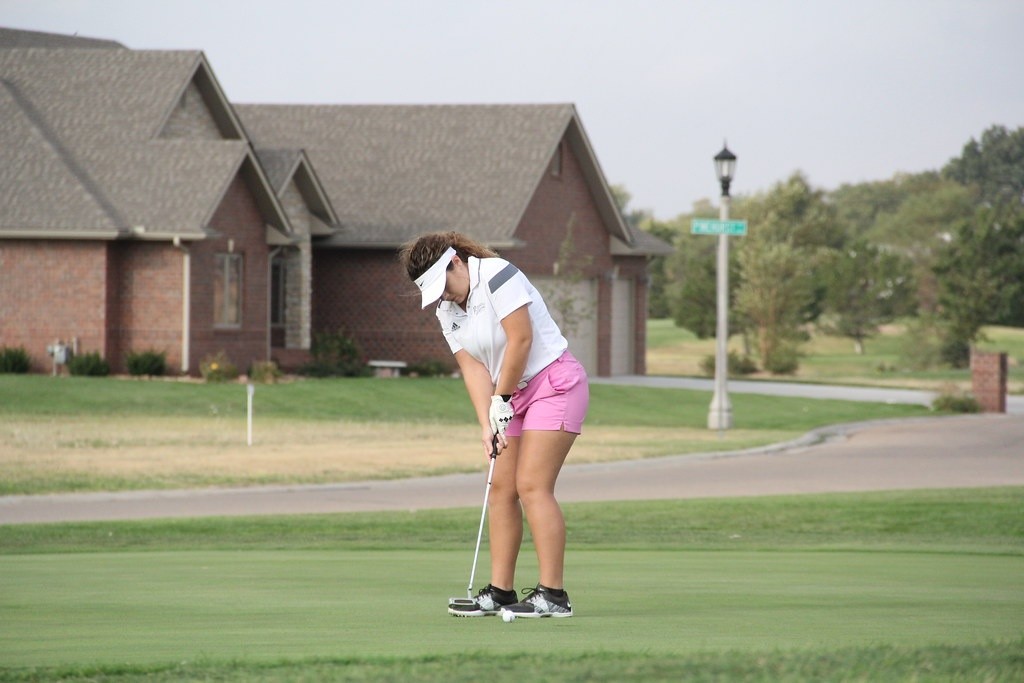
[407,232,590,618]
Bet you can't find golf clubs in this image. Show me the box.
[449,429,499,606]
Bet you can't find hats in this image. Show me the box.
[410,247,457,309]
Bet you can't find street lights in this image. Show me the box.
[706,138,735,432]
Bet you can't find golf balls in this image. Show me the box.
[502,610,515,623]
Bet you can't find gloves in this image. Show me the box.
[491,394,514,443]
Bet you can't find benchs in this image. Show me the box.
[367,360,408,379]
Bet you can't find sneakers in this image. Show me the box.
[500,584,574,620]
[447,584,519,617]
